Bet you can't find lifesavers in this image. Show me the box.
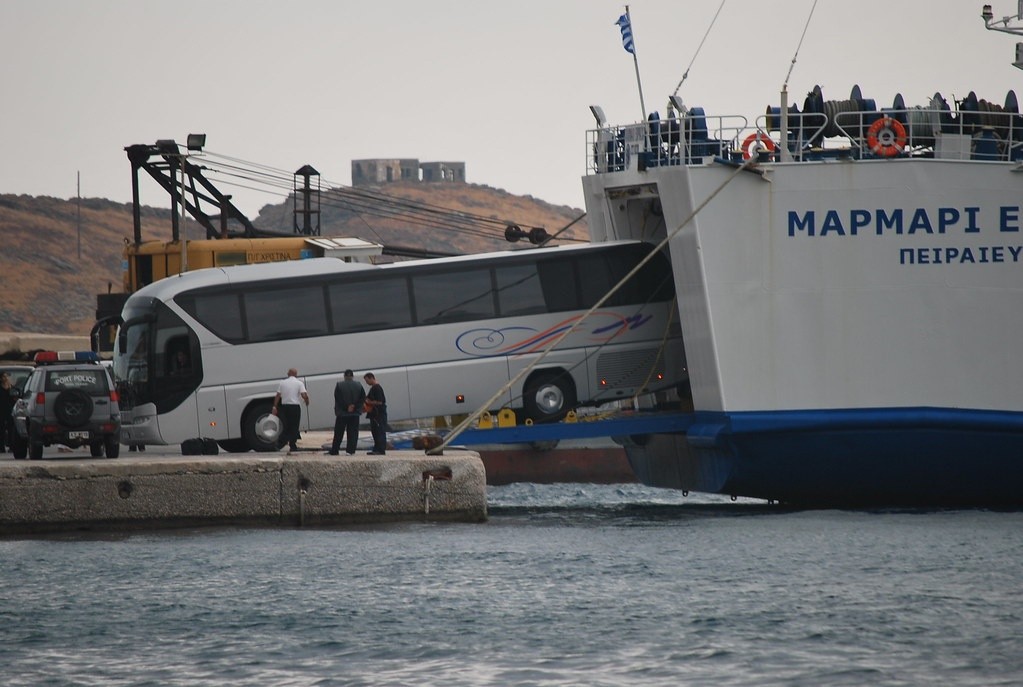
[741,133,775,160]
[867,116,907,157]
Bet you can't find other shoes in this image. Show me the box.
[346,452,351,456]
[367,451,383,455]
[139,448,145,452]
[127,450,136,452]
[323,452,339,455]
[289,448,301,452]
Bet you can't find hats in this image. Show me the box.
[345,369,353,376]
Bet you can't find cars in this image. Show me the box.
[2,362,36,410]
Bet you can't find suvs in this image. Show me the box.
[10,349,121,459]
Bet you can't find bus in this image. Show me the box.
[92,237,692,454]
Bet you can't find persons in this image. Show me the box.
[329,369,366,455]
[272,368,309,452]
[127,445,145,452]
[364,372,387,455]
[0,369,18,453]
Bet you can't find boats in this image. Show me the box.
[571,2,1022,522]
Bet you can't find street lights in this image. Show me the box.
[153,134,208,273]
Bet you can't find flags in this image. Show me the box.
[614,13,636,55]
